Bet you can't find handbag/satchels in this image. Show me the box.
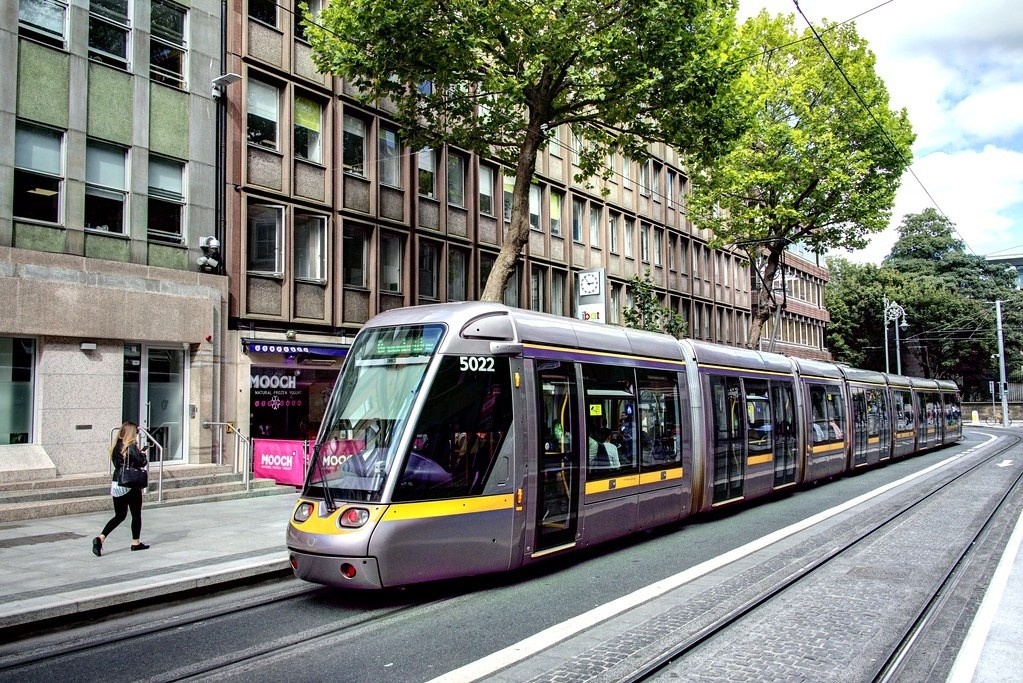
[118,446,148,490]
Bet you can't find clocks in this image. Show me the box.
[580,271,600,297]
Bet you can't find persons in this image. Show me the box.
[92,422,150,557]
[587,411,633,474]
[828,417,842,440]
[812,416,824,442]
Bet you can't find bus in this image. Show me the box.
[286,300,960,588]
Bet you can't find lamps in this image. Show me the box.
[81,343,96,350]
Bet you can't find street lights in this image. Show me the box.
[882,294,909,376]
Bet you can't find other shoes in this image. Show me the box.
[93,537,102,556]
[131,543,149,551]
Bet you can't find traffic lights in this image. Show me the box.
[996,384,999,392]
[1004,383,1007,390]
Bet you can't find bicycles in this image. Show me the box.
[985,408,1012,427]
